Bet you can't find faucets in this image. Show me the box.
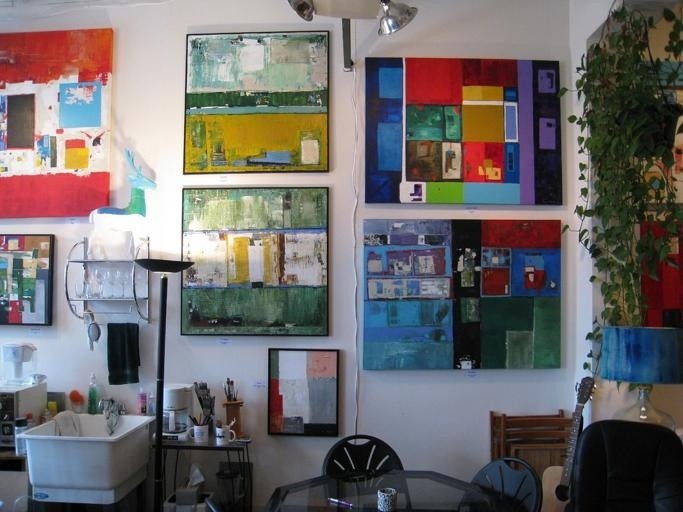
[98,398,115,411]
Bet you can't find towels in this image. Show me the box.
[105,322,140,386]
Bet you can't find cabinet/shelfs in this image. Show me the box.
[63,235,151,327]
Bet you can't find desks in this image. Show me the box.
[151,434,253,512]
[265,468,525,509]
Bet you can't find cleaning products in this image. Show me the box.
[134,386,147,415]
[148,393,157,414]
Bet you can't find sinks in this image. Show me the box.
[17,410,155,504]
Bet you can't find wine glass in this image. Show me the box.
[78,268,134,300]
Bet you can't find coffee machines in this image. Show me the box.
[156,382,194,441]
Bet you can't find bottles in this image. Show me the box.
[87,371,99,415]
[12,400,57,456]
[136,388,156,416]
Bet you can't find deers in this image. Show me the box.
[86,147,157,261]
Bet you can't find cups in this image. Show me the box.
[190,424,209,443]
[215,425,236,446]
[376,488,399,512]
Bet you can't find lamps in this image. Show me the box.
[286,0,418,74]
[596,322,681,430]
[135,253,194,510]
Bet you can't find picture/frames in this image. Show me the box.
[0,232,58,328]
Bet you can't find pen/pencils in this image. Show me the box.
[327,497,353,508]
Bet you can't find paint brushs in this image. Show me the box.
[223,377,238,401]
[189,413,211,426]
[194,381,216,415]
